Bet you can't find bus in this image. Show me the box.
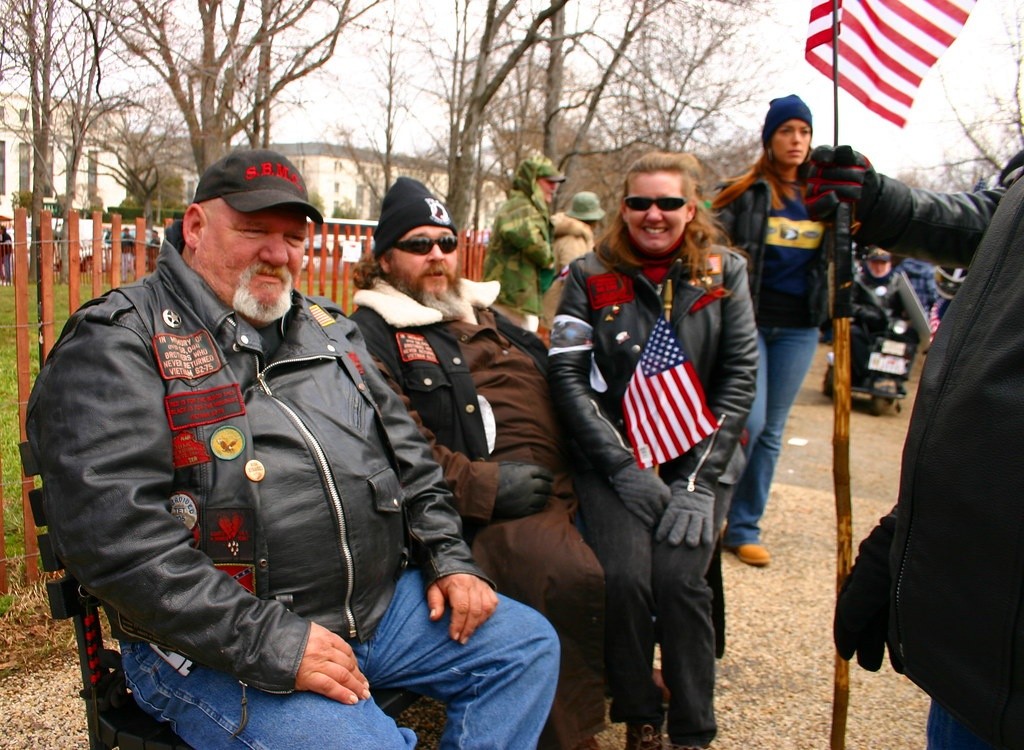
[301,218,381,258]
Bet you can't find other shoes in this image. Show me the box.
[624,720,663,750]
[895,383,907,396]
[732,544,769,565]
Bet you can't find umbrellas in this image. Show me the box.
[0,215,12,222]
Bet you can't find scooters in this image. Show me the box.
[822,285,924,417]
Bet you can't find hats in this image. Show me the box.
[564,190,606,220]
[536,157,567,184]
[373,177,458,260]
[864,244,890,263]
[193,150,324,226]
[761,94,814,144]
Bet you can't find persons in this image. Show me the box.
[845,241,919,394]
[797,141,1024,750]
[145,229,161,272]
[540,147,760,750]
[481,145,567,336]
[710,92,838,578]
[342,177,666,750]
[537,190,608,356]
[120,228,137,283]
[0,225,12,286]
[26,148,560,750]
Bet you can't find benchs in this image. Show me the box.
[17,440,423,750]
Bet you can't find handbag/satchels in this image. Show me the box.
[833,507,904,670]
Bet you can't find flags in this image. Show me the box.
[807,0,977,127]
[623,310,717,469]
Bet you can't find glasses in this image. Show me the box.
[624,197,686,211]
[391,236,459,255]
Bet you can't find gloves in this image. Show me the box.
[653,479,716,546]
[489,458,553,519]
[803,144,881,224]
[612,461,672,531]
